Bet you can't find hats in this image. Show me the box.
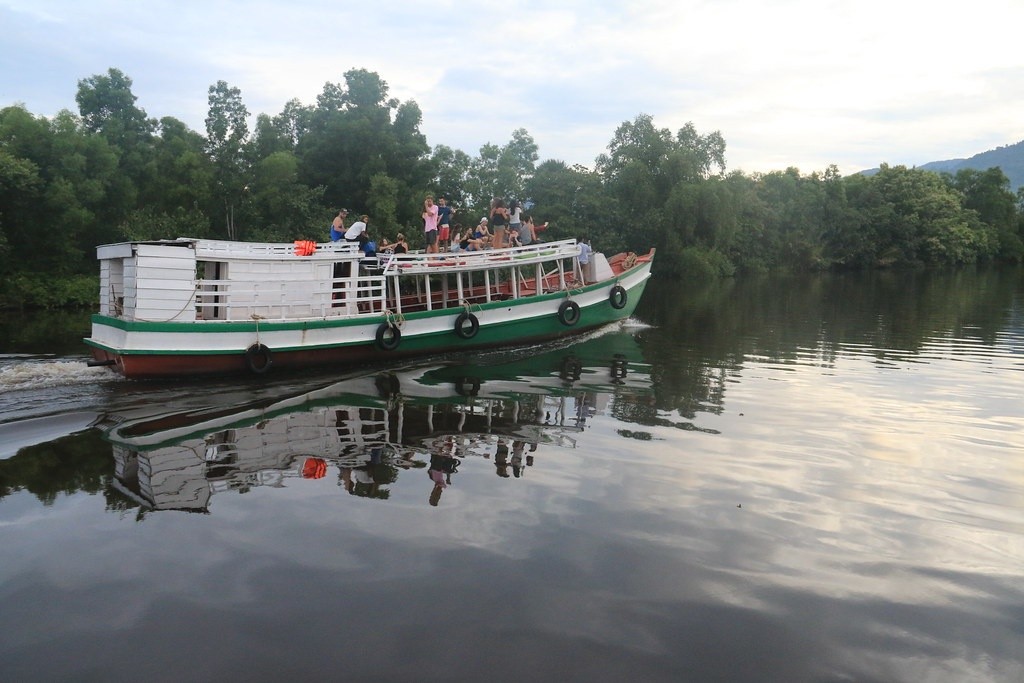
[340,208,349,214]
[480,217,489,223]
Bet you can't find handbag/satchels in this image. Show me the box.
[329,225,343,242]
[363,241,376,253]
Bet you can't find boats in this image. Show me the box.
[80,223,658,376]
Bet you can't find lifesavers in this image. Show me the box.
[455,374,481,397]
[375,372,401,400]
[557,301,581,326]
[610,285,628,309]
[454,312,479,338]
[376,322,402,351]
[247,343,272,374]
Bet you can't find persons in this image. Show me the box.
[331,195,550,261]
[576,235,592,286]
[338,433,537,506]
[576,400,589,431]
